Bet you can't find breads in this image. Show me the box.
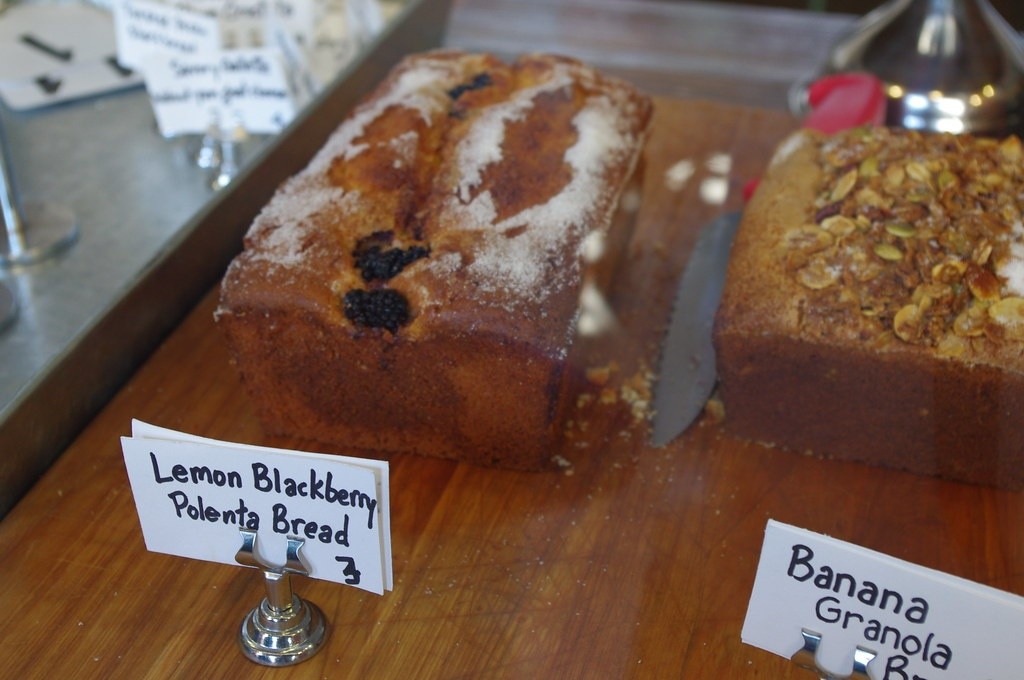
[214,48,658,472]
[707,128,1024,493]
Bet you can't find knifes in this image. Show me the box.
[648,74,888,444]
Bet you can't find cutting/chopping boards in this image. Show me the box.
[1,96,1024,680]
